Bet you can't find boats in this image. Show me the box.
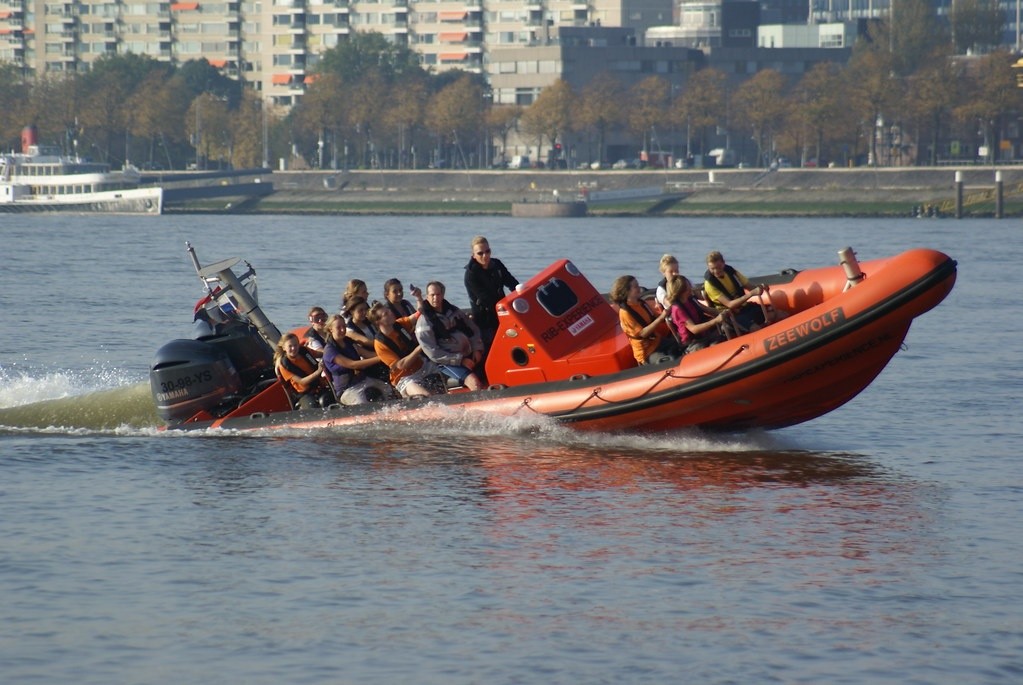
[0,124,164,216]
[148,235,960,438]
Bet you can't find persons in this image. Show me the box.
[464,237,519,354]
[705,251,788,332]
[274,278,451,409]
[553,187,559,203]
[416,282,489,391]
[610,255,730,366]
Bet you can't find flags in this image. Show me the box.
[229,279,258,312]
[194,286,235,322]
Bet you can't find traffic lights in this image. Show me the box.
[554,143,562,149]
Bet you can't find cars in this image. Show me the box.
[487,147,830,171]
[427,159,453,170]
[139,160,163,170]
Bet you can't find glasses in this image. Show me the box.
[312,314,328,323]
[476,249,490,255]
